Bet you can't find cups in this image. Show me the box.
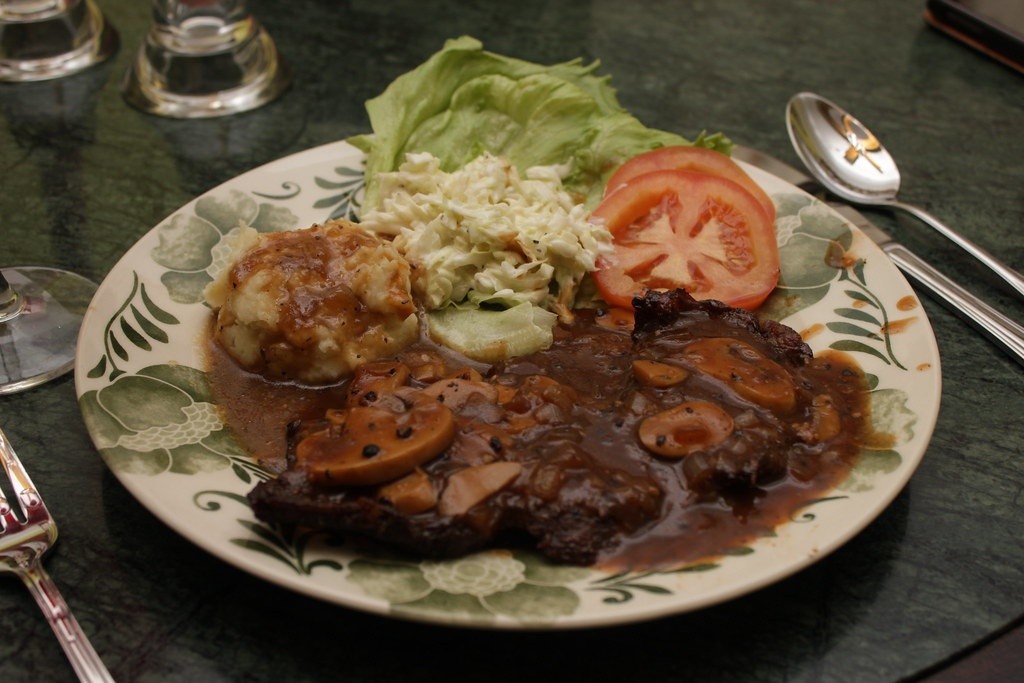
[119,0,287,116]
[0,0,111,83]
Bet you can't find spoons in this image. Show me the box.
[786,91,1023,297]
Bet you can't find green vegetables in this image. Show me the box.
[348,38,736,362]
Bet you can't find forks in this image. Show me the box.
[0,426,112,683]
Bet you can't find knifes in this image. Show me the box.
[683,134,1024,368]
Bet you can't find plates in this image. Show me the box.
[77,134,942,630]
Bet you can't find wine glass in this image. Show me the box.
[0,265,101,395]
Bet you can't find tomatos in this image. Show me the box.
[602,146,775,223]
[584,167,781,313]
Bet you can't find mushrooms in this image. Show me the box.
[678,336,798,409]
[639,402,736,461]
[294,364,458,488]
